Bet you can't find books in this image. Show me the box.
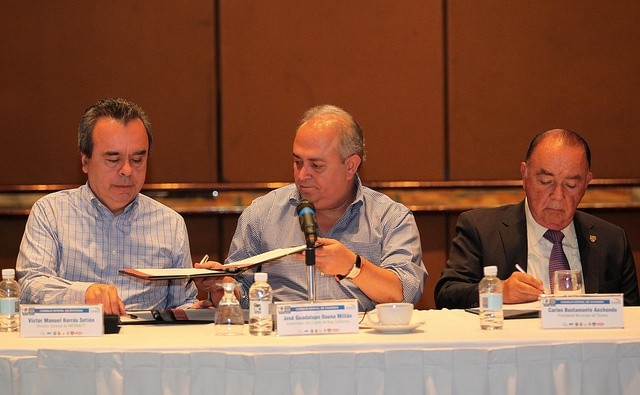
[464,300,540,318]
[118,310,249,324]
[119,242,323,280]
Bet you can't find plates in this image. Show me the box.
[366,322,426,334]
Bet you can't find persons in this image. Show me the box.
[434,128,640,310]
[16,99,214,315]
[195,105,428,310]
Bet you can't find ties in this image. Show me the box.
[543,229,573,294]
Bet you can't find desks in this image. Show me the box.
[0,307,640,395]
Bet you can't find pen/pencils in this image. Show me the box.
[515,263,546,295]
[185,254,209,288]
[124,313,138,319]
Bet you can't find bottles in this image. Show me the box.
[0,268,22,333]
[478,265,506,330]
[248,272,273,337]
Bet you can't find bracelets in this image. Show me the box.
[338,255,362,280]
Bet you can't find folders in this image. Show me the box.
[120,307,250,324]
[119,242,324,280]
[464,299,541,320]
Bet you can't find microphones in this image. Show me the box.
[296,198,318,248]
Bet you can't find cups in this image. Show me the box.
[552,269,584,299]
[368,302,413,324]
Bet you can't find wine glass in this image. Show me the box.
[211,280,246,336]
